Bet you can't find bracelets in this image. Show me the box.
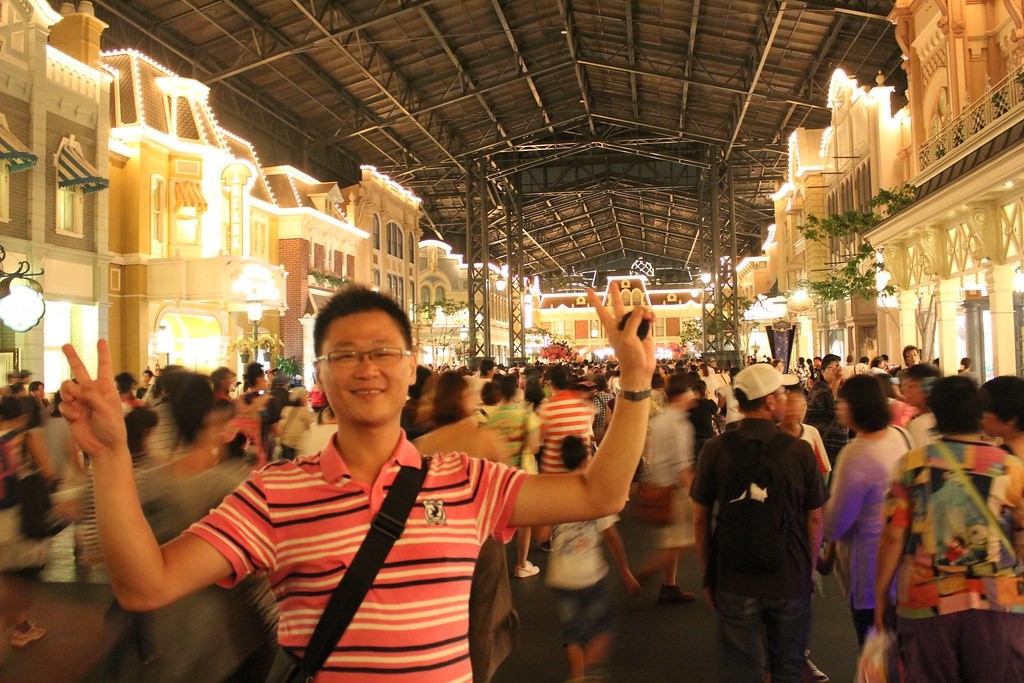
[615,382,652,401]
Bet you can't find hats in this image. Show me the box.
[274,371,290,386]
[732,363,799,400]
[288,386,309,402]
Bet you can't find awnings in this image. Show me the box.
[176,182,208,215]
[0,124,38,173]
[57,147,108,194]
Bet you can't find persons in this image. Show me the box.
[0,364,340,682]
[402,345,1024,683]
[59,281,656,683]
[688,363,829,683]
[893,345,919,400]
[874,376,1024,683]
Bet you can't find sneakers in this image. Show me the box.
[9,617,47,648]
[514,561,540,578]
[803,650,829,682]
[540,540,554,552]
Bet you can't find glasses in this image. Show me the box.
[772,388,793,397]
[312,346,414,369]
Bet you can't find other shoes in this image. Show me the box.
[657,585,694,604]
[628,570,645,602]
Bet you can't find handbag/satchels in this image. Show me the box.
[853,626,899,683]
[634,487,671,529]
[19,473,72,540]
